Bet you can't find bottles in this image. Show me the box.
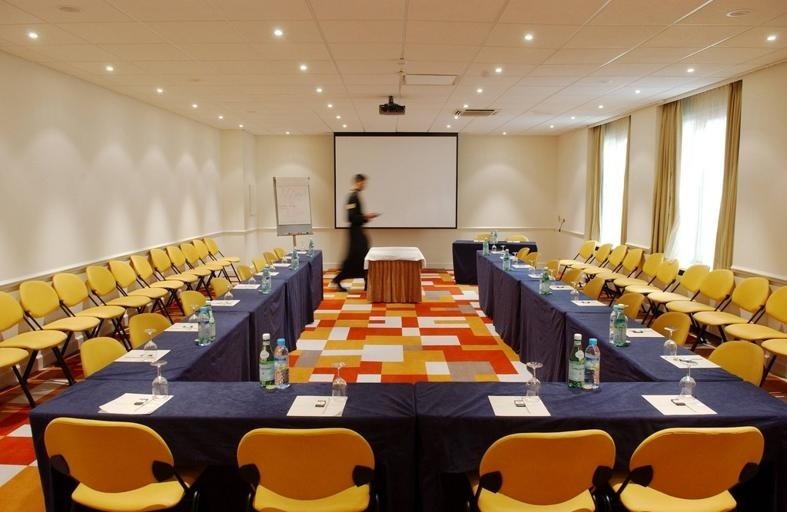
[197,306,212,347]
[308,238,314,258]
[584,337,602,390]
[259,333,274,390]
[567,333,585,390]
[204,300,216,342]
[273,338,291,390]
[610,304,617,344]
[613,304,626,347]
[260,263,272,294]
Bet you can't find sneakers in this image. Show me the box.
[327,280,348,292]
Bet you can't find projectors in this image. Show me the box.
[378,103,405,116]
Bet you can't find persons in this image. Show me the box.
[327,172,382,292]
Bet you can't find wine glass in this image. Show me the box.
[150,360,169,401]
[663,327,679,359]
[679,359,697,402]
[570,280,579,303]
[526,360,543,402]
[143,327,159,357]
[223,283,234,305]
[330,362,349,404]
[248,245,300,285]
[481,237,554,296]
[187,305,199,330]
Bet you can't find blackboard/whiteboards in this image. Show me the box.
[273,176,312,236]
